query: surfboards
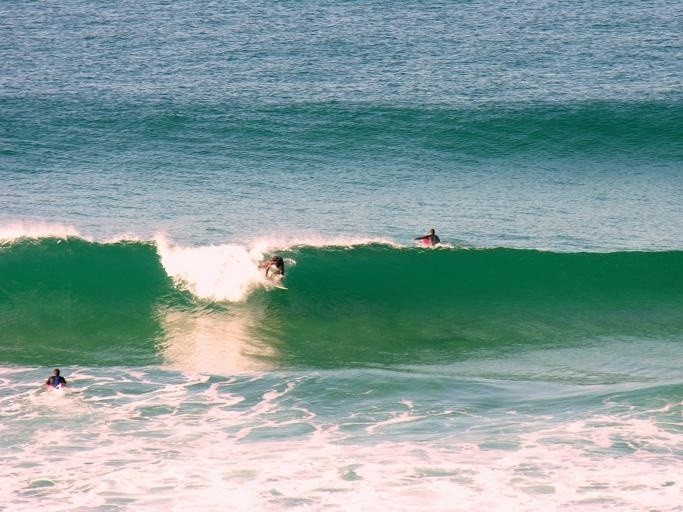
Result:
[270,280,288,290]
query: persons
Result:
[261,256,285,280]
[414,228,440,248]
[46,369,65,391]
